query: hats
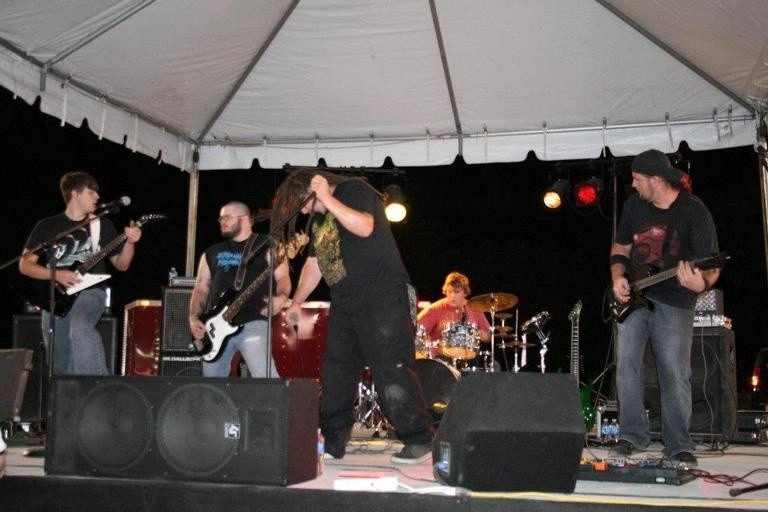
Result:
[630,149,690,184]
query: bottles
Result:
[167,265,177,286]
[317,427,325,478]
[602,418,619,438]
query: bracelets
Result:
[703,279,708,293]
[279,291,288,297]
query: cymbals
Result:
[494,332,516,337]
[496,325,513,332]
[468,293,519,313]
[495,313,512,318]
[497,341,535,347]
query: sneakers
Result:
[609,438,646,457]
[389,441,434,465]
[673,451,698,467]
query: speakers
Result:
[431,373,587,495]
[44,373,320,486]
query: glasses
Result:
[217,215,246,222]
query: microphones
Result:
[96,196,131,209]
[289,312,299,334]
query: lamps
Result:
[283,165,408,227]
[543,152,691,402]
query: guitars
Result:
[568,301,595,431]
[25,214,167,318]
[607,251,734,323]
[192,230,310,363]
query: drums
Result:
[443,323,481,360]
[414,324,430,359]
[407,283,417,331]
[413,358,461,428]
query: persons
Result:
[18,171,143,376]
[189,201,292,379]
[270,167,437,464]
[416,271,502,373]
[611,150,721,464]
